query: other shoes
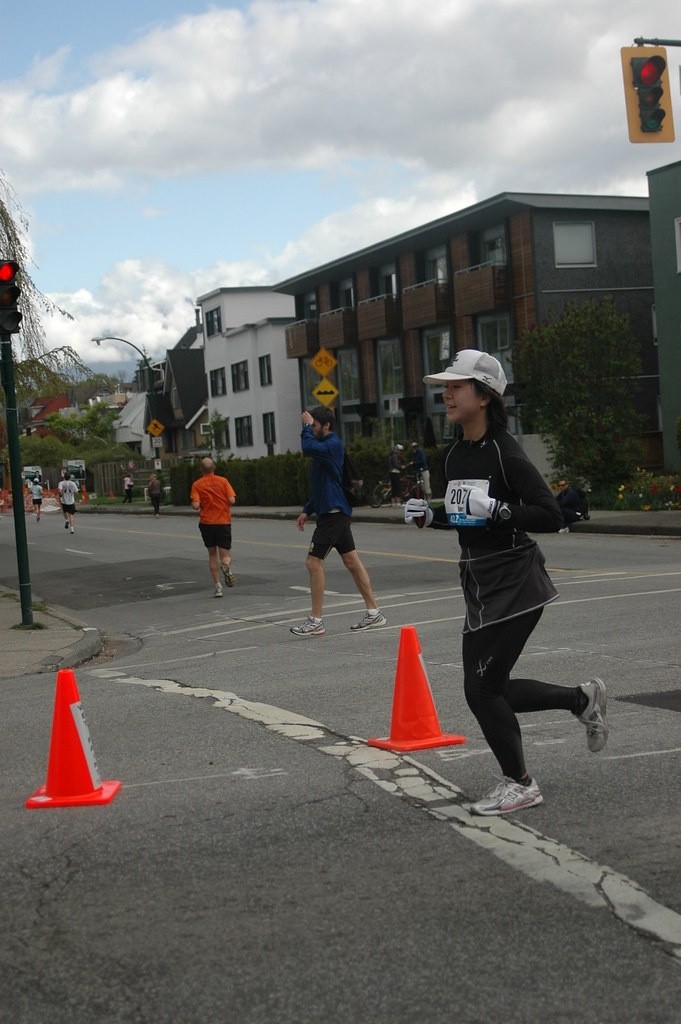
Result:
[221,561,237,588]
[214,590,224,597]
[37,514,41,522]
[71,529,74,533]
[390,503,401,507]
[65,520,69,529]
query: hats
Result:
[411,442,418,447]
[423,350,508,397]
[396,444,404,451]
[34,477,39,482]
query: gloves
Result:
[405,498,433,531]
[460,485,497,518]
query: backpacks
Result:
[332,445,362,507]
[570,486,589,521]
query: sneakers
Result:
[558,527,570,534]
[470,768,543,815]
[290,615,326,636]
[571,677,609,753]
[351,609,386,631]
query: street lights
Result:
[91,335,156,468]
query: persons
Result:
[57,472,79,534]
[555,478,582,535]
[404,354,610,816]
[148,473,161,517]
[28,478,46,522]
[290,408,386,636]
[77,465,85,478]
[407,442,432,503]
[32,471,41,482]
[190,457,236,598]
[388,443,410,508]
[122,473,134,503]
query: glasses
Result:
[558,484,566,487]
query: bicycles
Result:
[365,468,429,508]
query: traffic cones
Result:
[23,667,125,810]
[367,625,468,753]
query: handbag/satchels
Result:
[147,488,152,497]
[128,484,134,488]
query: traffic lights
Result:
[0,257,24,340]
[620,45,676,144]
